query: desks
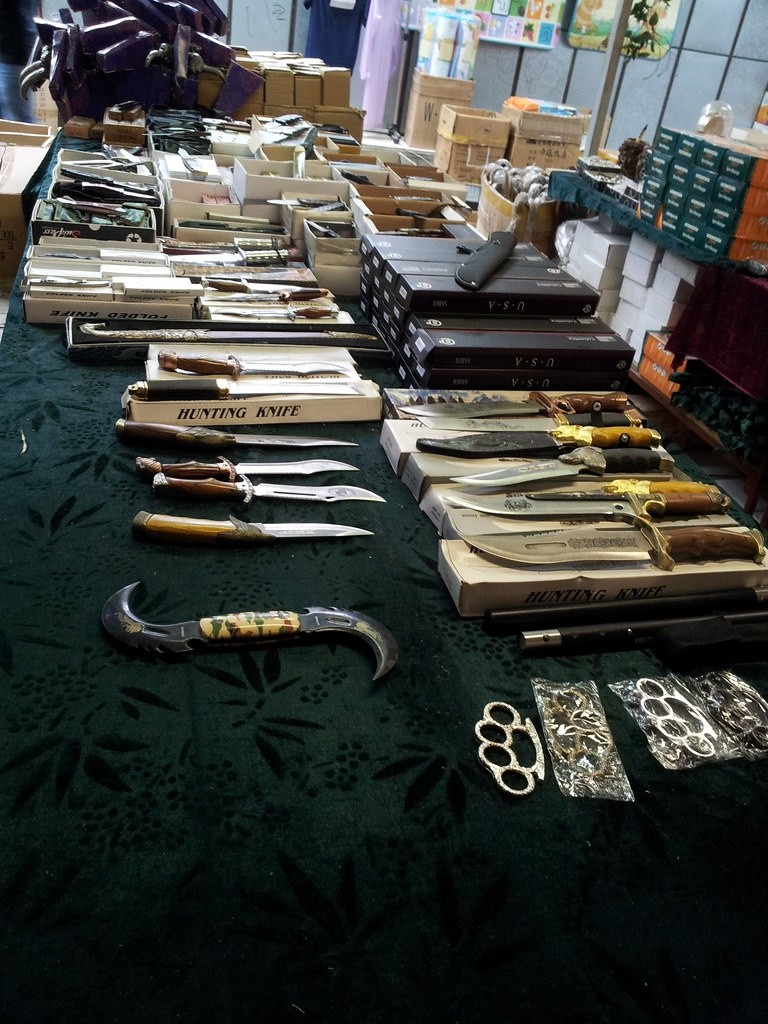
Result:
[0,129,768,1024]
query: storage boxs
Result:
[379,388,768,617]
[0,119,63,297]
[405,67,475,151]
[197,46,368,145]
[19,104,636,427]
[436,97,768,400]
